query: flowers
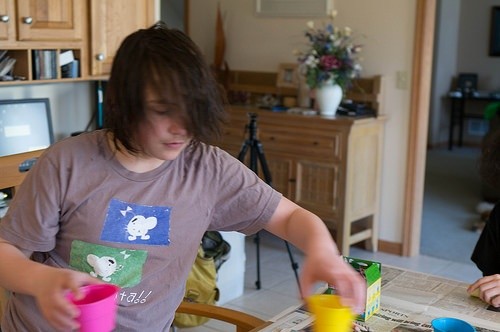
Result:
[292,10,364,91]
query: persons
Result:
[0,21,368,332]
[467,199,500,308]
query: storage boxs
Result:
[211,228,247,307]
[327,255,382,322]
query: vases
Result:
[314,71,343,120]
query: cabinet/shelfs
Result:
[0,0,157,86]
[204,70,387,258]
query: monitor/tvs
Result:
[0,98,54,158]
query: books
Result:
[33,50,56,79]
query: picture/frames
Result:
[276,63,300,89]
[254,0,329,19]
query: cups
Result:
[305,294,358,332]
[432,317,476,332]
[66,283,121,332]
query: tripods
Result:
[235,113,304,300]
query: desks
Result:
[247,263,500,332]
[447,93,500,152]
